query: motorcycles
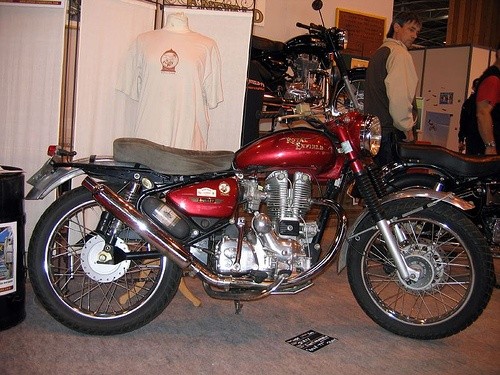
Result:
[247,0,366,118]
[25,111,496,341]
[346,134,500,259]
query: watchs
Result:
[485,141,497,147]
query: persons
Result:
[458,78,485,157]
[364,9,422,164]
[116,12,224,150]
[475,46,500,157]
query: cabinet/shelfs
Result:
[406,43,498,154]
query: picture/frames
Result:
[335,7,388,61]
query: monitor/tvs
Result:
[337,54,371,72]
